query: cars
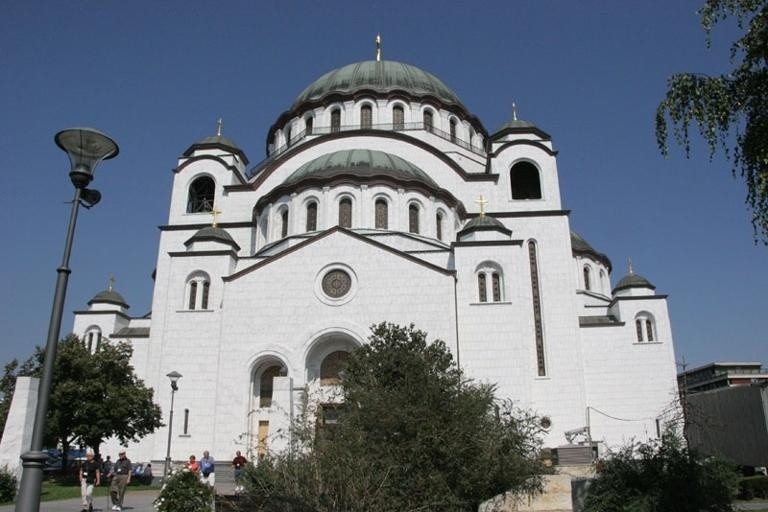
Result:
[46,449,153,485]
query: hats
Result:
[119,450,126,455]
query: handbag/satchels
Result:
[86,475,94,483]
[203,473,209,476]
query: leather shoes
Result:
[81,506,93,512]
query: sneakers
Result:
[235,485,244,491]
[112,505,120,512]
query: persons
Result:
[102,455,151,479]
[107,451,132,511]
[199,450,216,491]
[78,447,100,512]
[232,449,248,491]
[186,455,200,472]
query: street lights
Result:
[15,126,120,512]
[164,370,183,475]
